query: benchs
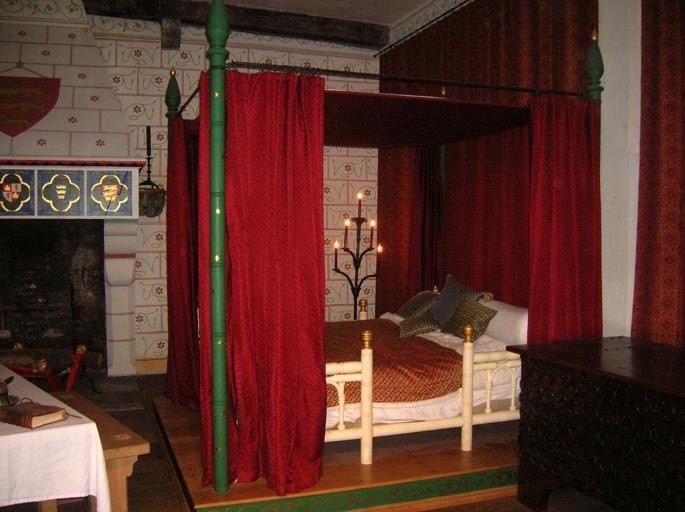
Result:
[38,389,151,512]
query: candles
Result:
[333,192,383,274]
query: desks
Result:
[1,363,112,512]
[506,335,683,511]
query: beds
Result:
[321,298,529,465]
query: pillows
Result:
[396,274,498,340]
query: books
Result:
[1,402,70,429]
